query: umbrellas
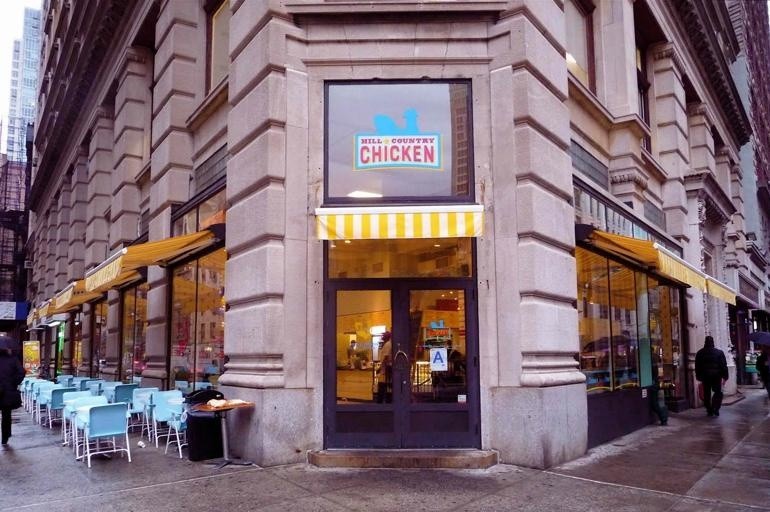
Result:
[0,336,19,349]
[583,335,679,353]
[745,330,770,346]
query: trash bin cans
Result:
[183,389,224,462]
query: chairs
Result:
[20,375,188,468]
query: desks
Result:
[193,402,255,469]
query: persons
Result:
[0,349,26,444]
[446,340,456,360]
[347,340,357,364]
[756,345,770,400]
[591,347,661,378]
[694,336,729,416]
[376,337,392,374]
